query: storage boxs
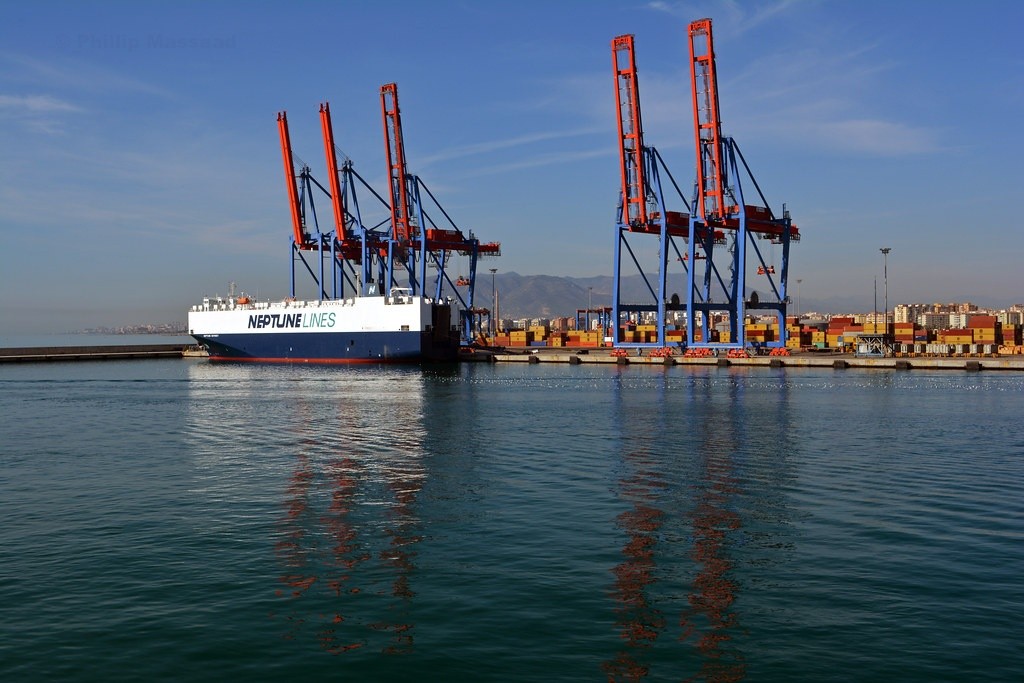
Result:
[475,317,1017,356]
[648,197,772,229]
[428,229,463,244]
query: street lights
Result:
[796,279,802,319]
[490,268,498,334]
[880,248,891,334]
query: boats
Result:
[188,287,461,364]
[182,345,210,357]
